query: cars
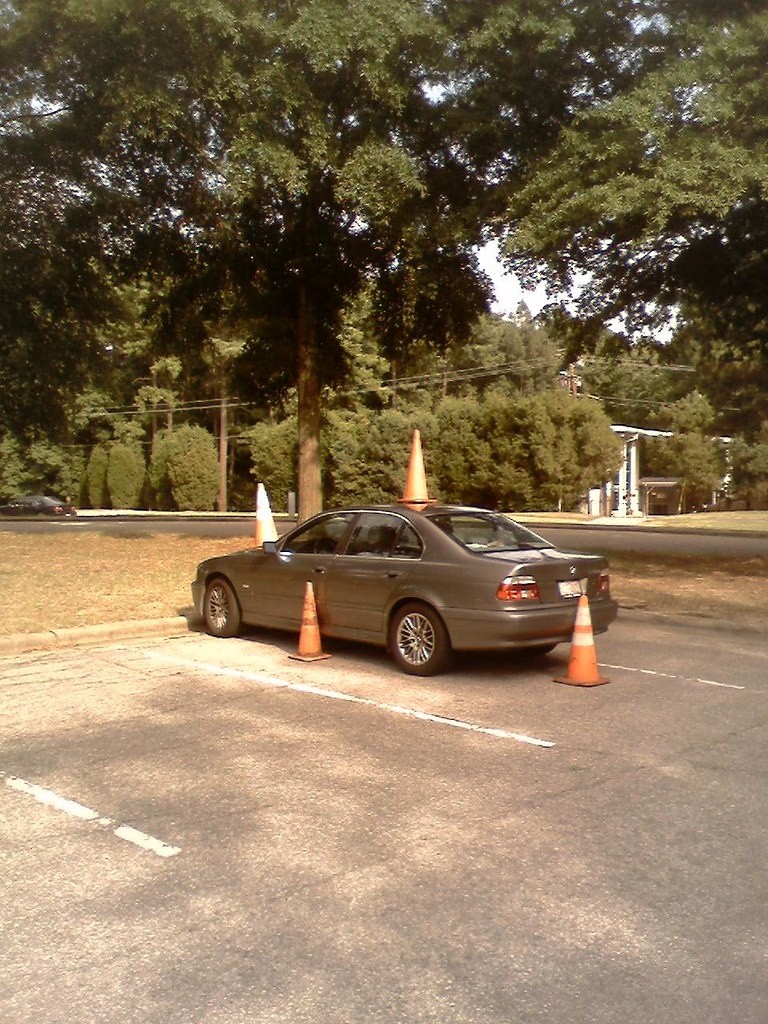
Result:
[190,504,619,676]
[0,496,77,517]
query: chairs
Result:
[367,526,397,548]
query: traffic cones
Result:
[552,594,612,687]
[287,581,333,662]
[395,429,438,503]
[253,483,280,549]
